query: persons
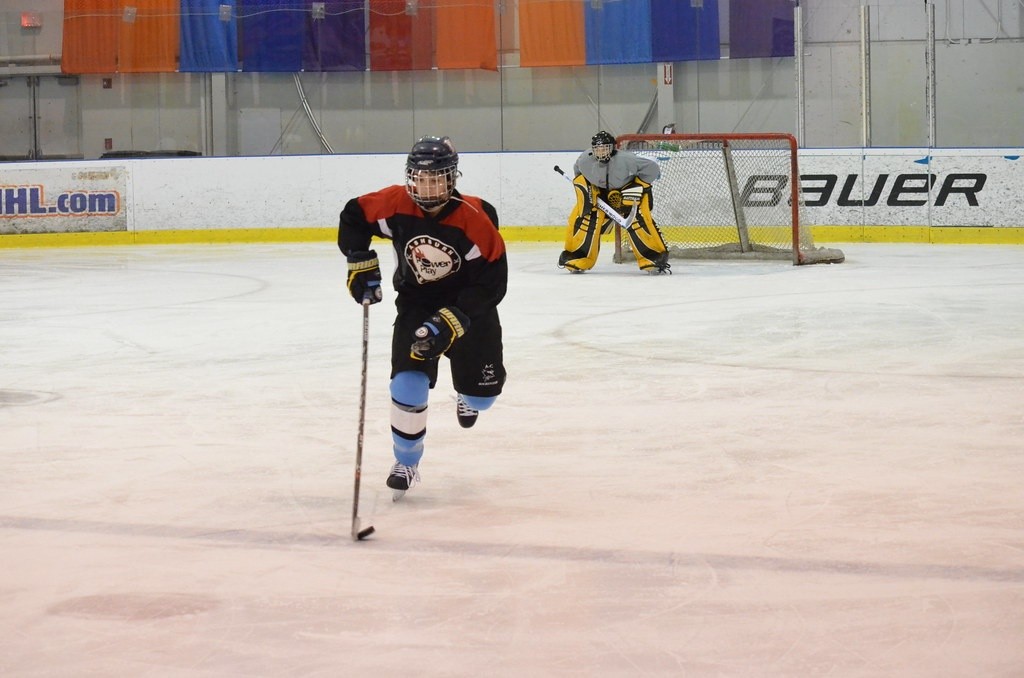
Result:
[338,136,508,501]
[559,131,670,275]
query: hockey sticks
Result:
[351,292,375,541]
[554,164,637,228]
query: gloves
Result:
[410,304,471,360]
[346,250,382,305]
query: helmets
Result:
[591,130,617,163]
[405,135,462,212]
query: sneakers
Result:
[385,460,421,502]
[456,392,479,429]
